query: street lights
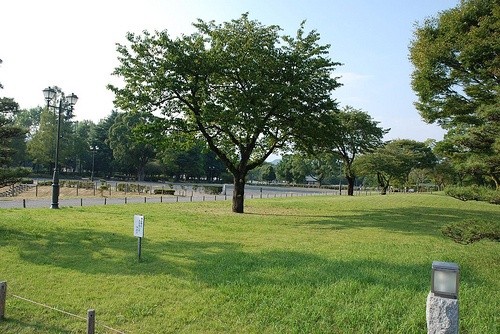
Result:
[42,87,79,210]
